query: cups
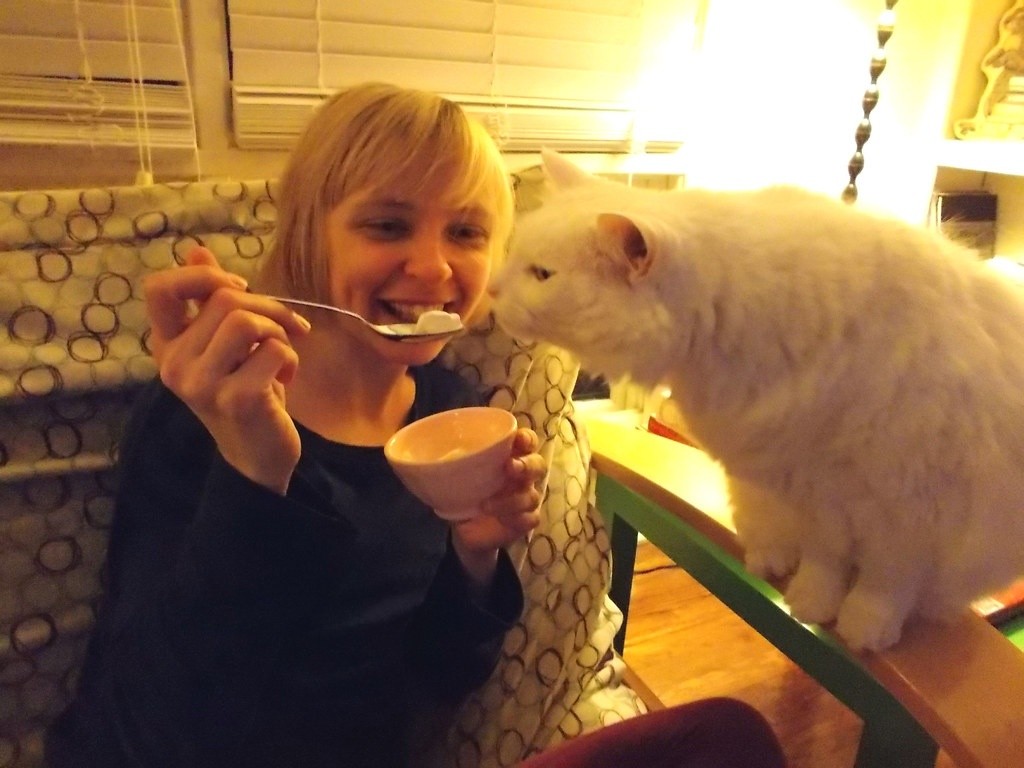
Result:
[936,190,998,261]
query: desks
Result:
[595,471,1024,768]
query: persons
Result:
[47,77,785,767]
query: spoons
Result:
[250,292,466,345]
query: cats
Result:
[488,145,1024,655]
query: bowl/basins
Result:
[384,407,518,523]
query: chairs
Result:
[0,170,1024,768]
